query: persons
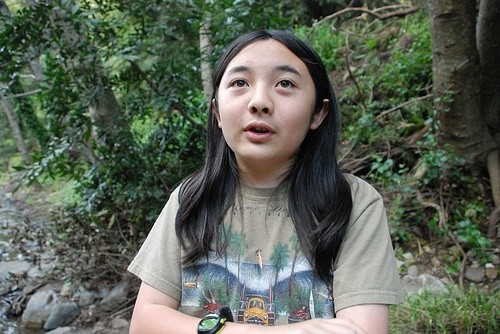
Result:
[126,30,405,334]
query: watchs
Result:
[197,310,227,334]
